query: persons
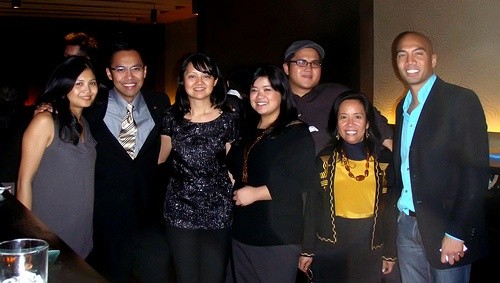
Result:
[298,90,397,283]
[16,32,314,283]
[282,40,393,160]
[393,30,490,283]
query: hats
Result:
[285,40,324,61]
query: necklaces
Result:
[242,122,274,183]
[340,144,370,181]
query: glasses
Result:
[290,58,321,68]
[111,64,145,73]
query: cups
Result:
[0,238,49,283]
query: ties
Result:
[117,104,137,160]
